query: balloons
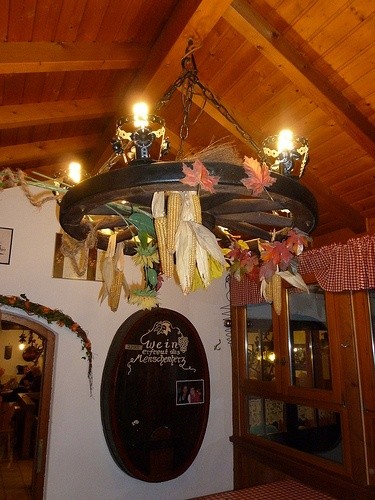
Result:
[23,346,38,362]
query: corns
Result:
[106,188,285,317]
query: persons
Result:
[0,356,44,461]
[189,387,200,403]
[178,385,191,404]
[197,387,203,399]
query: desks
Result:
[199,479,337,500]
